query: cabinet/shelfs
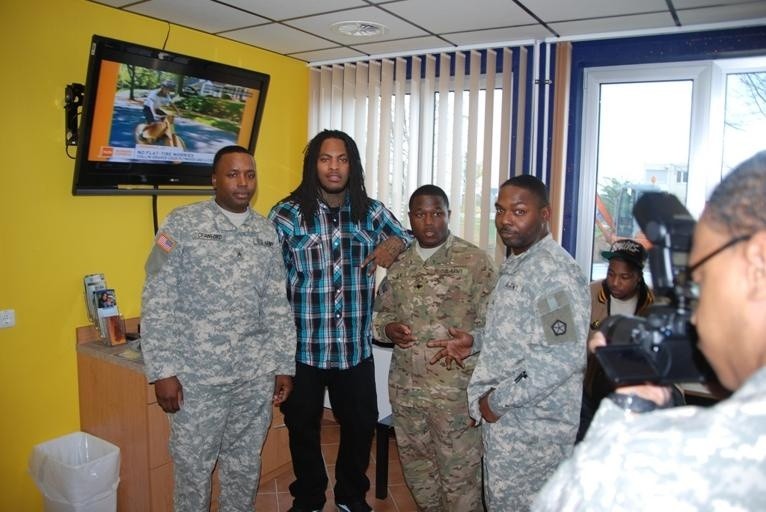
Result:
[76,317,292,512]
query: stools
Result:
[375,412,394,500]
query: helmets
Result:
[160,79,176,90]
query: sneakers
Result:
[334,493,373,512]
[286,503,323,512]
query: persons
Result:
[143,81,176,126]
[424,174,592,512]
[140,146,298,512]
[531,152,766,512]
[99,292,115,308]
[370,184,499,512]
[267,129,413,511]
[574,240,655,451]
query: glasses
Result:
[671,233,755,300]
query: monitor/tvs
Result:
[72,34,270,196]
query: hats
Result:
[600,239,650,267]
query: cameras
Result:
[593,192,707,387]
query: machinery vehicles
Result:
[595,182,663,263]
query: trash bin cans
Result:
[33,431,120,512]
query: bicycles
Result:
[131,107,187,166]
